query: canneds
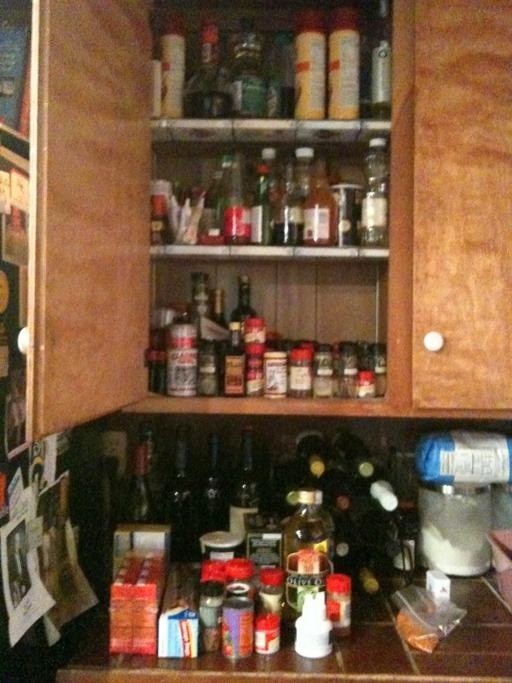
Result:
[223,583,254,660]
[263,342,314,399]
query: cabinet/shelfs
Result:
[22,0,510,444]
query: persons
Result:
[8,530,28,607]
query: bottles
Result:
[293,590,336,660]
[286,424,401,638]
[153,0,393,119]
[151,270,265,396]
[150,193,172,245]
[199,579,225,654]
[230,425,265,515]
[202,137,389,248]
[162,424,199,561]
[264,337,388,399]
[258,570,283,623]
[197,428,230,532]
[124,419,163,522]
[257,439,289,515]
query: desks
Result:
[53,562,512,680]
